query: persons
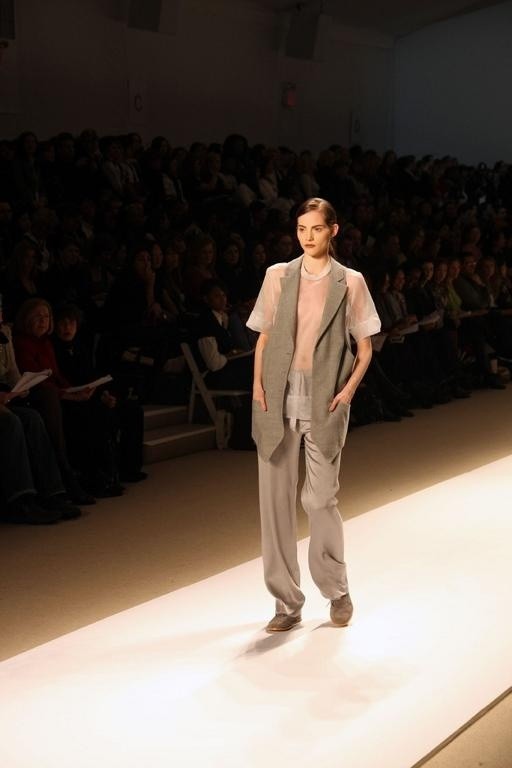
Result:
[0,129,512,528]
[242,195,383,633]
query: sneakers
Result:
[5,462,147,527]
[266,612,303,631]
[346,353,512,424]
[329,590,354,627]
